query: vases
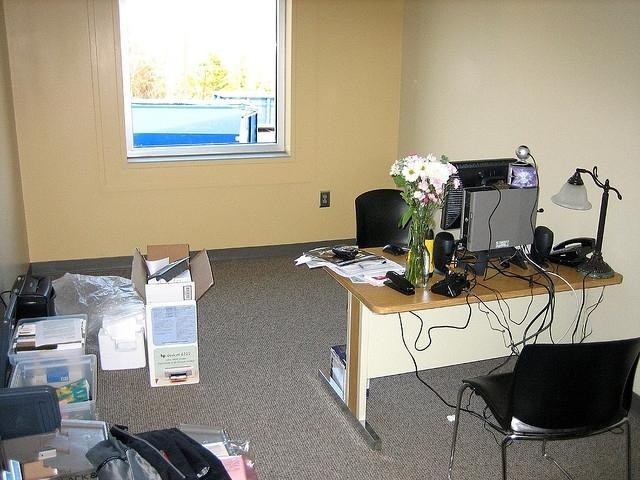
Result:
[404,233,426,288]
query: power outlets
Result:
[320,191,331,207]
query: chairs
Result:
[445,336,640,479]
[353,189,414,253]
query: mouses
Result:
[383,244,404,256]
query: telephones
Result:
[548,238,595,266]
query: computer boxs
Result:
[461,185,538,253]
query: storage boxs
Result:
[178,422,237,460]
[130,241,215,388]
[10,355,101,421]
[7,313,88,360]
[0,420,112,479]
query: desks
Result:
[311,242,624,453]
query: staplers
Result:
[384,271,415,295]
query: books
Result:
[296,245,405,289]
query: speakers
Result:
[532,225,553,269]
[432,232,454,274]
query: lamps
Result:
[550,162,621,279]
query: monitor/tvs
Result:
[440,158,534,276]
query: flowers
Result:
[387,151,459,282]
